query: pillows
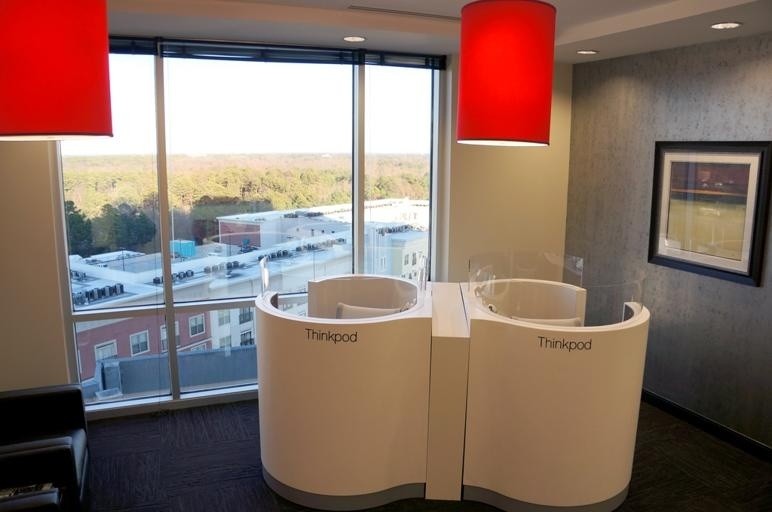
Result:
[487,303,583,327]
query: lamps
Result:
[455,0,557,148]
[0,1,115,142]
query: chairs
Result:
[0,382,90,511]
[334,301,409,319]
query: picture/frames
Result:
[646,138,771,287]
